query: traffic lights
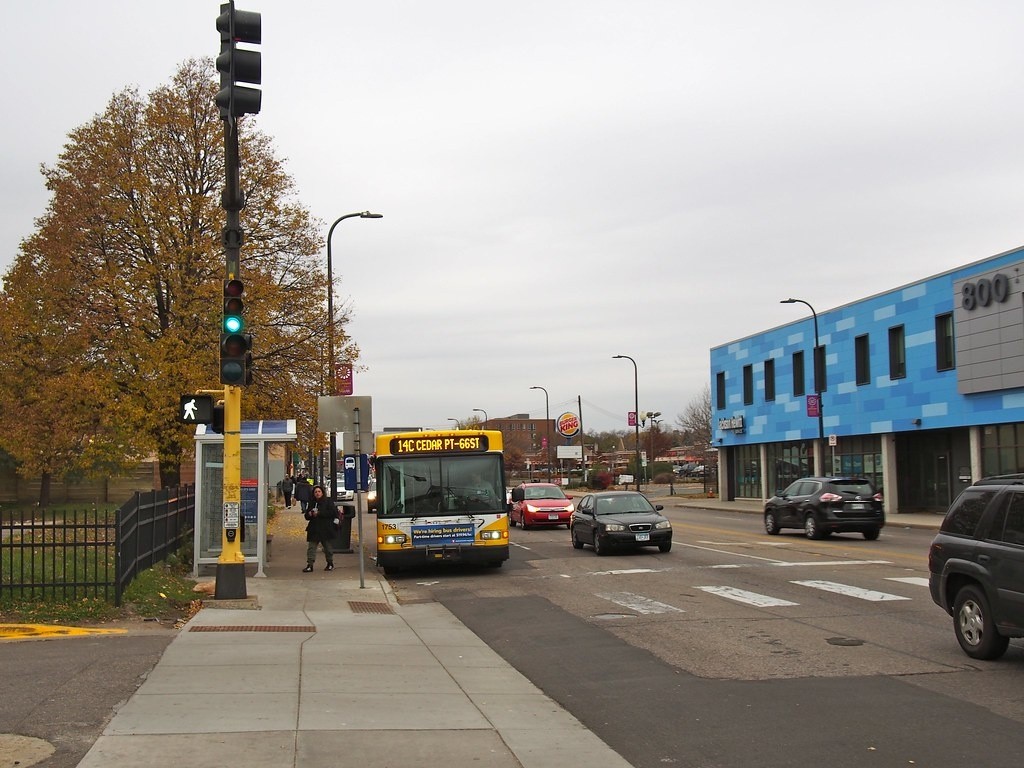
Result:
[218,333,253,385]
[222,279,244,334]
[178,394,213,424]
[214,0,262,116]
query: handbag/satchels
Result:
[292,498,296,506]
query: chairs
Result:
[527,489,532,498]
[597,500,610,513]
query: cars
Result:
[571,491,673,556]
[319,471,377,501]
[507,481,574,530]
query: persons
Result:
[462,471,497,510]
[420,462,459,511]
[282,473,294,510]
[303,486,339,572]
[290,474,311,513]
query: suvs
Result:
[764,477,886,540]
[928,473,1024,660]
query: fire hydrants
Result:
[669,483,676,495]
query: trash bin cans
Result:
[322,502,355,554]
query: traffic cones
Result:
[707,487,714,498]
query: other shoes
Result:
[288,506,291,509]
[302,510,306,513]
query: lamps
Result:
[715,437,721,442]
[911,419,922,425]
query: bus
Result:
[373,429,525,575]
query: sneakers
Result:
[325,562,333,572]
[302,564,313,572]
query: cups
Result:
[313,508,318,517]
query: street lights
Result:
[647,411,661,481]
[529,386,550,484]
[473,408,488,429]
[780,298,825,477]
[327,211,383,503]
[611,354,647,492]
